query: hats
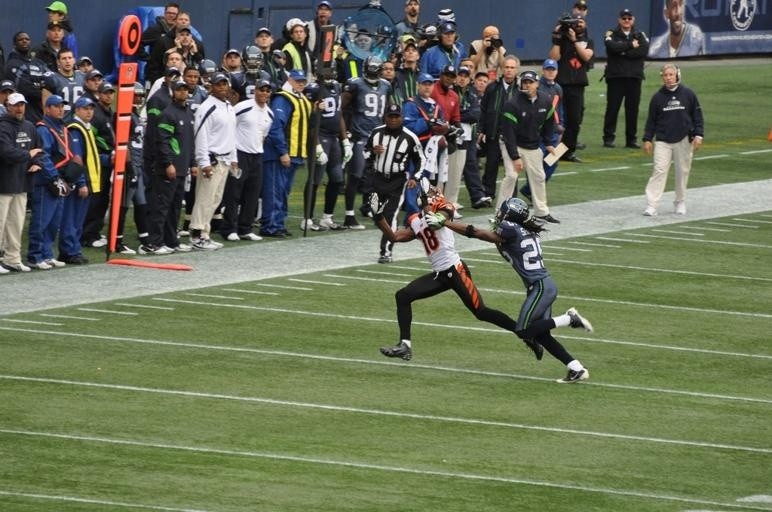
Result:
[571,14,586,21]
[0,1,116,108]
[574,0,589,10]
[383,104,402,117]
[520,70,539,83]
[542,59,559,70]
[162,0,490,110]
[619,8,634,17]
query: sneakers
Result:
[1,261,31,272]
[3,266,10,274]
[566,307,593,332]
[560,155,582,163]
[471,195,495,209]
[300,219,328,231]
[557,366,591,384]
[57,253,89,265]
[227,232,240,241]
[43,258,66,267]
[379,341,414,361]
[176,227,190,239]
[520,189,532,202]
[318,216,348,231]
[576,143,587,150]
[523,339,544,360]
[239,232,263,240]
[626,140,641,149]
[25,260,53,270]
[674,201,686,215]
[189,238,224,251]
[453,202,466,220]
[115,245,137,255]
[172,243,192,252]
[604,141,615,148]
[91,235,108,247]
[137,244,176,255]
[531,214,561,225]
[642,204,659,216]
[344,215,366,230]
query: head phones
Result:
[516,70,539,88]
[659,63,681,81]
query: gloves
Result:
[425,210,446,230]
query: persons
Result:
[603,9,650,149]
[368,185,544,361]
[1,0,595,275]
[426,199,593,384]
[650,0,706,58]
[642,64,704,216]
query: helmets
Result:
[418,184,447,208]
[497,197,530,224]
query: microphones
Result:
[670,81,680,86]
[518,87,533,93]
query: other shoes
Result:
[367,210,374,223]
[257,229,293,237]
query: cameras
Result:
[486,38,504,47]
